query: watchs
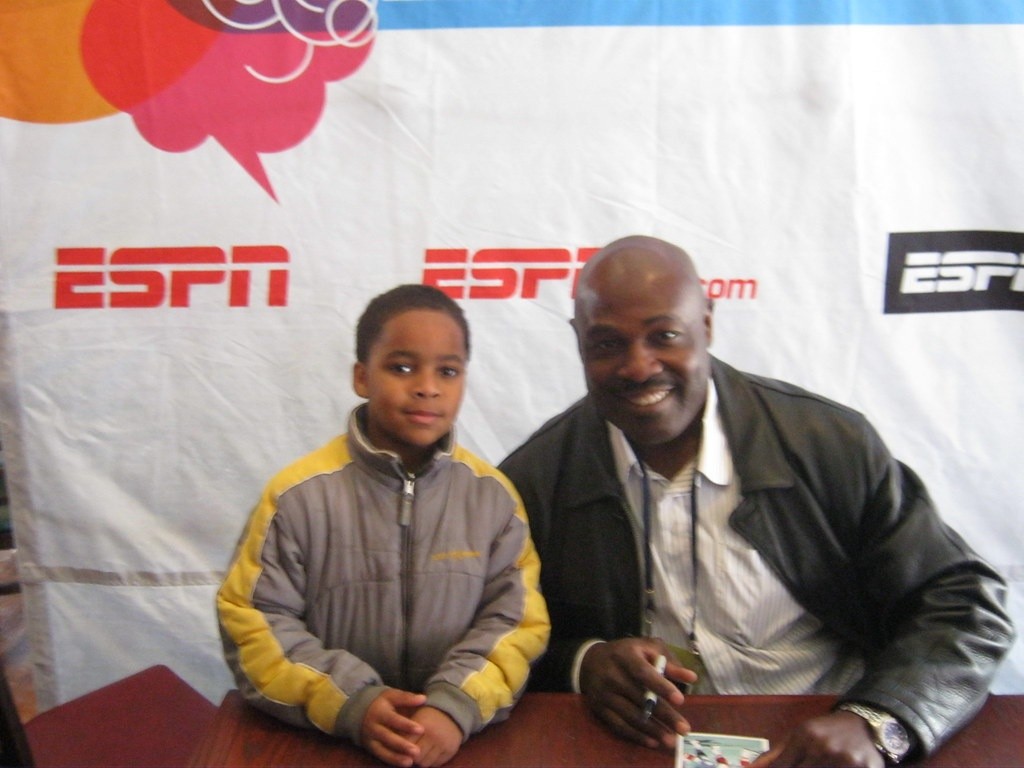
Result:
[836,701,915,768]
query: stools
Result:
[20,665,218,768]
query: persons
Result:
[215,285,551,767]
[495,234,1014,768]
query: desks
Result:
[193,689,1023,768]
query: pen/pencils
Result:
[637,656,665,728]
